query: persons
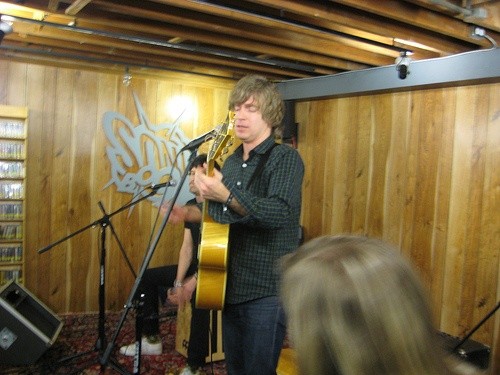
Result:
[118,74,306,375]
[275,233,457,375]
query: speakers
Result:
[0,279,64,370]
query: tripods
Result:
[39,190,156,375]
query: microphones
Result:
[151,179,176,188]
[182,125,223,151]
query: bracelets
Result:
[173,280,184,288]
[224,191,233,207]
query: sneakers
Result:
[120,336,162,355]
[180,363,201,375]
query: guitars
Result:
[193,109,239,313]
